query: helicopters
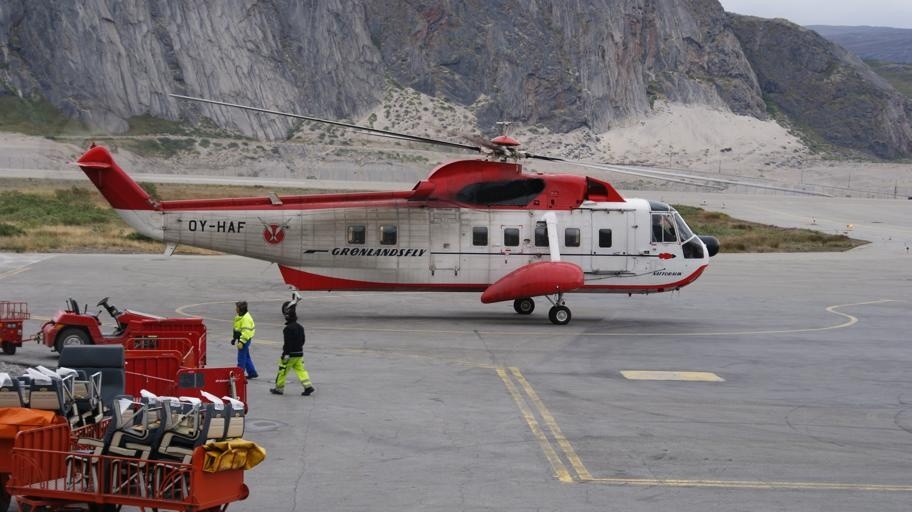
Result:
[66,94,831,324]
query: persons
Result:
[270,309,314,395]
[652,215,674,242]
[231,301,258,379]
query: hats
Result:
[285,310,297,321]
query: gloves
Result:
[237,341,243,349]
[283,355,290,361]
[230,339,235,345]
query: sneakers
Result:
[269,387,284,395]
[301,386,315,396]
[245,375,261,380]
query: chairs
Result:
[69,295,99,320]
[0,344,246,499]
